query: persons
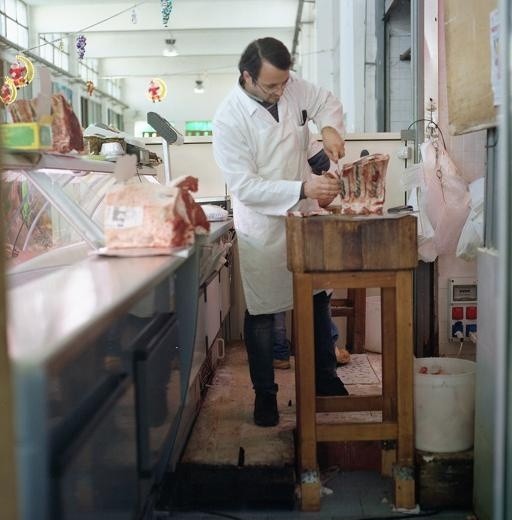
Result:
[210,34,355,426]
[6,53,36,89]
[146,78,167,104]
[0,76,19,105]
[272,125,363,370]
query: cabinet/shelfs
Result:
[1,152,158,294]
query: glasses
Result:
[253,73,294,95]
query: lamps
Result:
[193,74,205,98]
[162,29,180,57]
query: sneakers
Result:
[317,378,349,397]
[274,359,290,369]
[336,348,350,364]
[253,394,279,427]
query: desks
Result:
[290,267,419,511]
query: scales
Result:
[146,111,229,222]
